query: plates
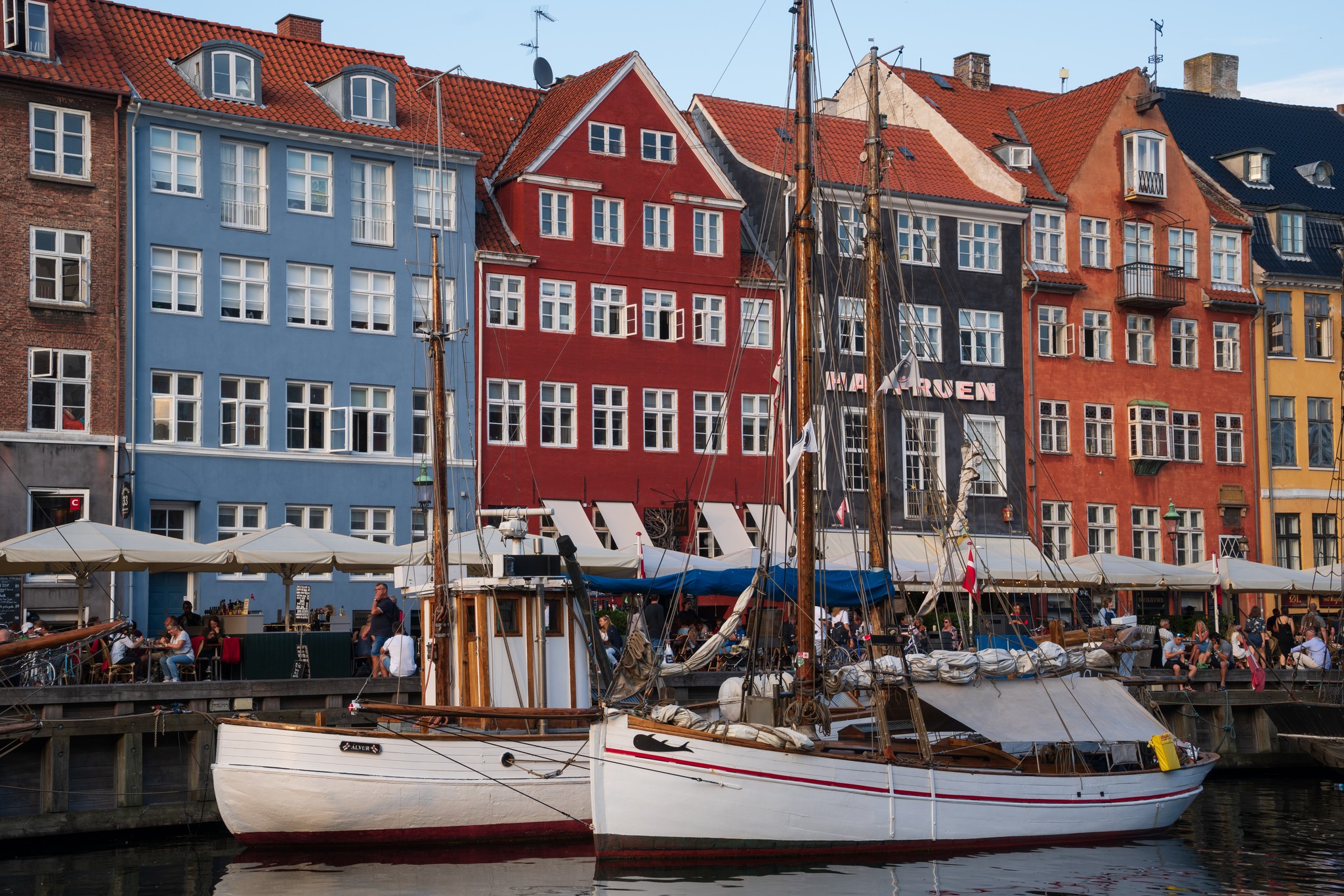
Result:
[153,645,165,647]
[138,645,147,647]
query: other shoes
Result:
[162,679,179,682]
[127,680,136,683]
[1285,665,1293,669]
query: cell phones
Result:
[1215,639,1220,645]
[1183,649,1187,652]
[209,632,214,636]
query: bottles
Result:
[737,634,740,640]
[326,612,331,622]
[289,611,292,623]
[703,628,707,640]
[742,634,744,639]
[791,658,794,671]
[735,628,737,640]
[693,629,696,640]
[340,606,345,616]
[309,607,327,623]
[208,599,244,615]
[339,608,341,616]
[312,614,320,631]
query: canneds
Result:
[933,625,936,631]
[1184,660,1188,666]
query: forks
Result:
[141,636,146,644]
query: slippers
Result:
[1218,685,1226,691]
[1180,689,1185,691]
[1187,689,1198,692]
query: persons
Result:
[900,615,927,654]
[1162,631,1198,692]
[0,601,224,687]
[1224,624,1246,669]
[1098,597,1115,626]
[1244,602,1344,670]
[323,583,405,677]
[1008,602,1028,624]
[627,592,744,672]
[780,604,872,666]
[1158,618,1174,648]
[1188,620,1209,668]
[1201,632,1235,691]
[593,614,622,673]
[380,621,417,677]
[942,616,959,651]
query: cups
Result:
[282,616,285,624]
[711,631,713,635]
[933,625,936,631]
[148,638,154,647]
[707,632,711,635]
[150,638,155,645]
[1183,635,1186,640]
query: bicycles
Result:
[903,617,929,655]
[826,625,873,666]
[950,619,976,651]
[0,624,83,687]
[1023,617,1089,636]
[793,618,851,670]
[588,615,625,673]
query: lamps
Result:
[426,642,438,660]
[1231,525,1251,553]
[1002,502,1014,522]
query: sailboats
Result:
[586,0,1221,866]
[207,63,1147,848]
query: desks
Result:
[202,643,223,682]
[263,622,331,632]
[135,643,172,683]
[695,639,709,646]
[1180,638,1197,662]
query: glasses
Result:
[943,621,949,624]
[374,588,385,592]
[1196,623,1204,626]
[1176,633,1184,637]
[167,627,174,632]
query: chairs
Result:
[98,638,135,685]
[671,633,729,672]
[210,636,245,681]
[1223,635,1344,669]
[144,659,165,683]
[410,636,421,676]
[174,635,206,682]
[84,640,118,685]
[1154,636,1169,669]
[349,628,372,677]
[758,636,794,672]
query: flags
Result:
[1241,634,1265,692]
[962,542,980,602]
[1211,550,1223,606]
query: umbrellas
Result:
[1052,549,1221,609]
[913,546,1099,615]
[165,522,423,632]
[1179,556,1344,633]
[710,546,858,571]
[398,525,642,579]
[821,550,964,584]
[0,519,230,685]
[584,541,748,578]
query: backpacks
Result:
[1303,615,1319,636]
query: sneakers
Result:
[205,667,212,673]
[207,674,211,678]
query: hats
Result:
[21,622,34,633]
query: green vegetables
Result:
[155,644,163,645]
[139,643,146,645]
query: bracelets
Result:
[1216,649,1220,653]
[1331,640,1334,642]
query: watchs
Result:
[1288,654,1292,657]
[373,598,379,602]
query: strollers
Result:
[720,636,754,671]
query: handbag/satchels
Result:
[662,643,674,663]
[835,621,845,630]
[158,650,178,658]
[124,650,136,657]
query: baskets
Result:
[832,626,849,645]
[597,624,608,641]
[966,625,980,639]
[915,631,929,647]
[961,639,976,648]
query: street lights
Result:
[411,458,433,541]
